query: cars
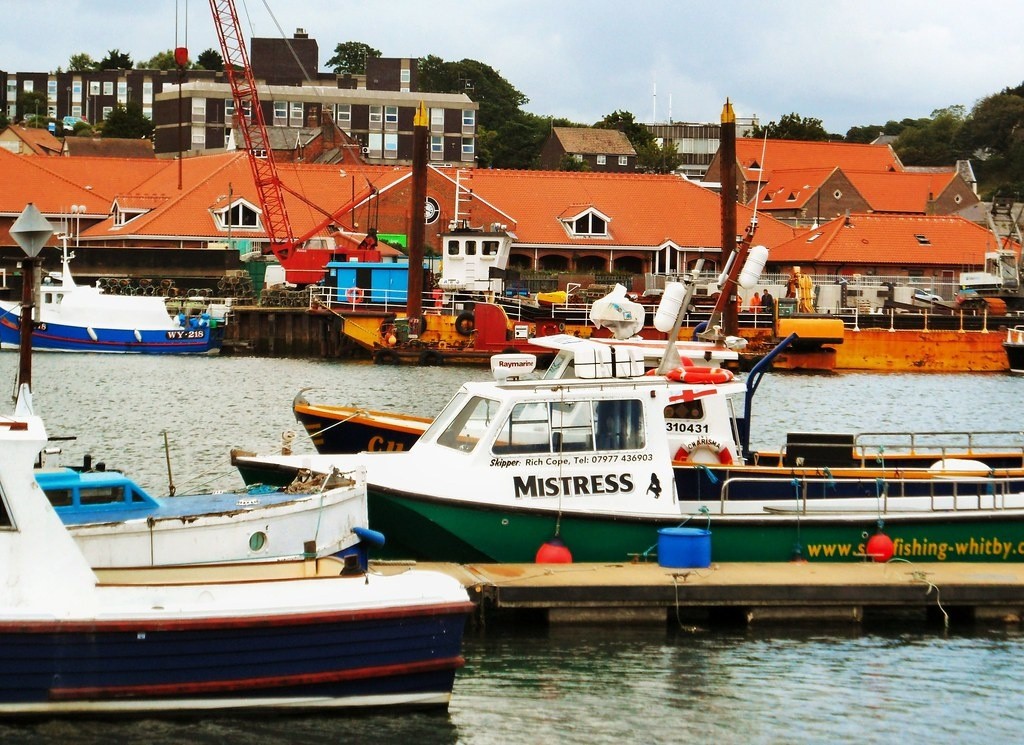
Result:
[912,287,945,301]
[62,117,90,127]
[48,122,73,133]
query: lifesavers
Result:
[346,286,363,303]
[673,435,735,466]
[454,313,477,337]
[668,365,735,384]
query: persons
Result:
[762,290,775,314]
[748,292,763,314]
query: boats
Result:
[229,372,1024,565]
[1002,325,1024,375]
[280,380,1024,492]
[0,291,231,357]
[0,204,478,719]
[586,332,740,378]
[31,442,372,576]
[28,223,103,300]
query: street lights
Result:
[70,204,85,246]
[715,96,742,340]
[397,96,430,325]
[34,97,40,129]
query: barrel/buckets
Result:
[657,507,712,568]
